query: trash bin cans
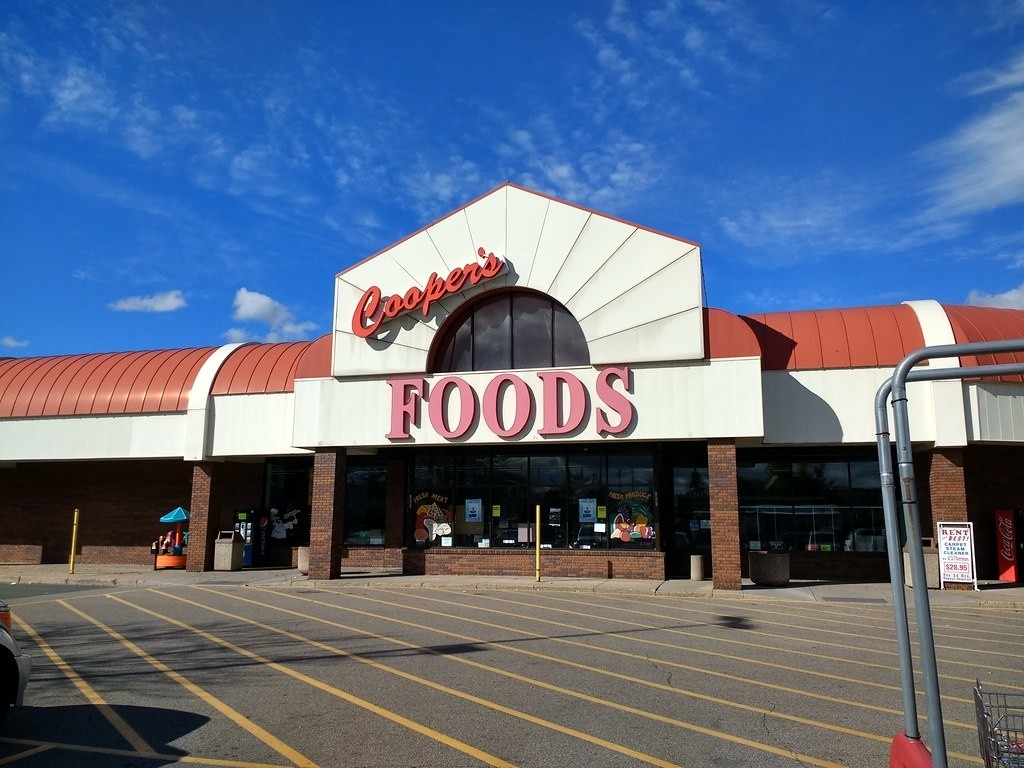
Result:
[211,529,245,571]
[901,537,939,589]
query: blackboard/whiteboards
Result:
[936,521,978,583]
